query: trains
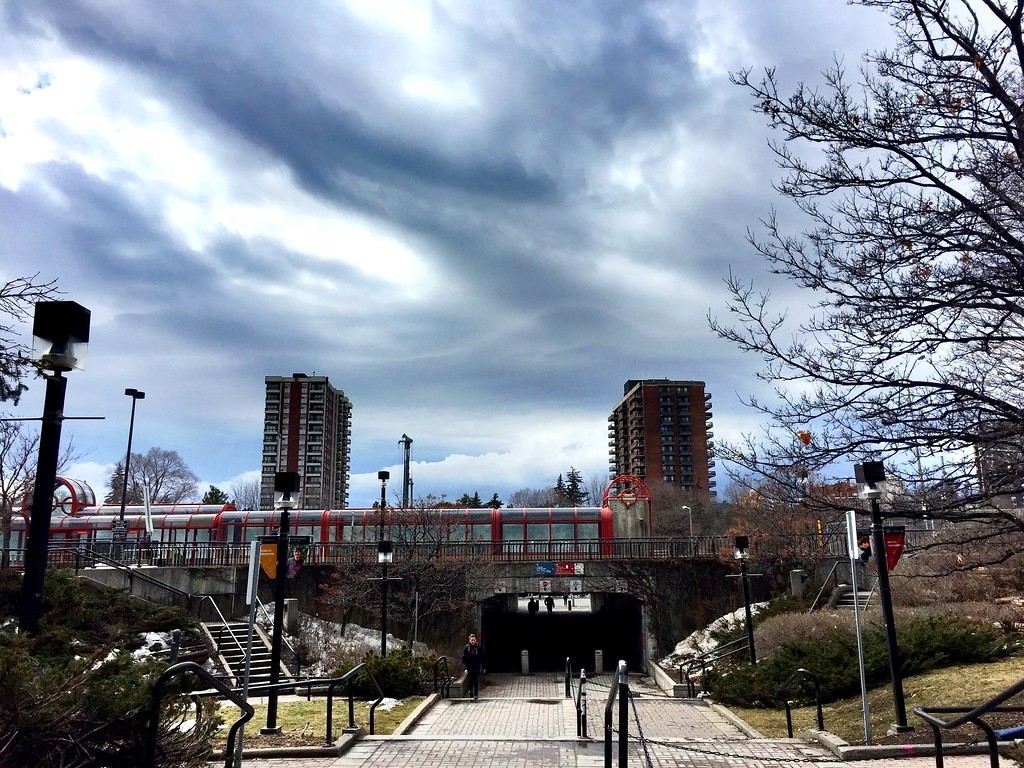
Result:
[0,502,613,571]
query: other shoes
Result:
[474,690,478,699]
[470,691,473,698]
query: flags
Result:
[884,525,905,569]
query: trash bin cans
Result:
[254,534,280,563]
[288,535,314,565]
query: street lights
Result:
[855,460,916,734]
[378,471,391,541]
[681,505,694,556]
[378,540,394,658]
[259,471,302,734]
[17,299,93,637]
[733,535,757,664]
[118,388,146,521]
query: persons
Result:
[288,545,303,578]
[461,633,486,702]
[545,594,554,613]
[861,537,872,559]
[563,592,569,604]
[528,597,538,614]
